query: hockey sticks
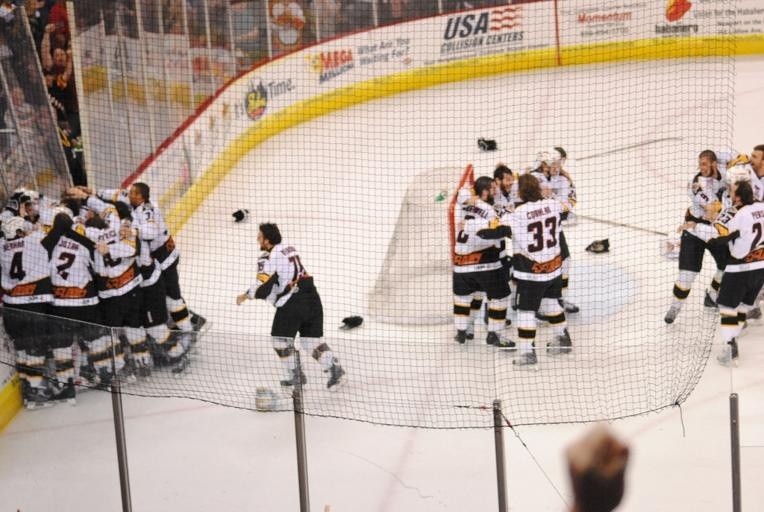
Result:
[575,136,682,161]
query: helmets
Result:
[722,166,751,184]
[534,148,560,178]
[42,206,74,228]
[4,216,33,240]
[7,188,43,216]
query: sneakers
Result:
[665,306,679,323]
[512,352,536,364]
[704,293,718,308]
[280,368,307,385]
[717,338,738,361]
[564,302,578,314]
[544,335,570,352]
[79,366,153,385]
[455,314,514,348]
[327,366,345,388]
[173,314,207,371]
[23,383,75,402]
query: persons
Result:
[208,0,514,79]
[236,223,346,393]
[0,0,208,410]
[449,146,580,366]
[565,426,628,512]
[663,145,764,367]
